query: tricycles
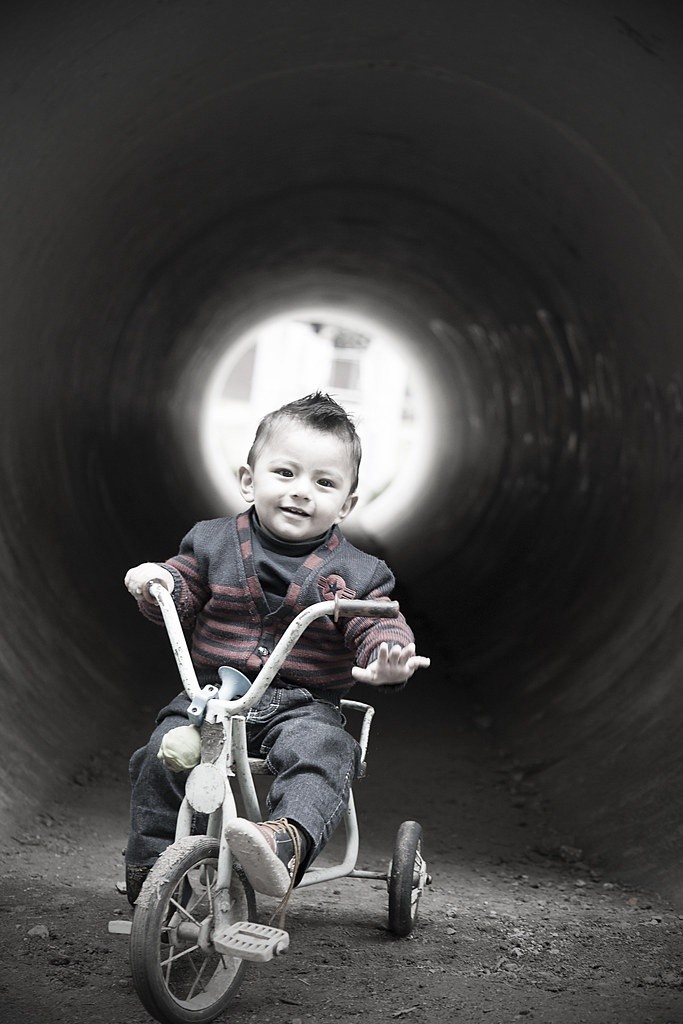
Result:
[105,573,433,1024]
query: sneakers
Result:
[160,919,180,948]
[225,817,306,897]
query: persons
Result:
[124,387,429,940]
